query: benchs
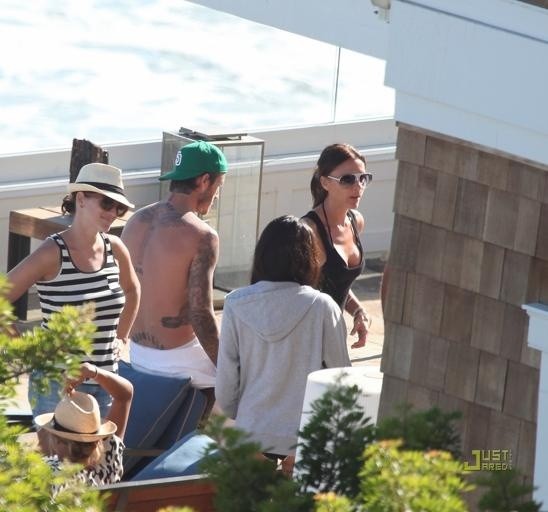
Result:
[85,474,223,512]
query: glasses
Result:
[327,173,373,186]
[85,192,128,217]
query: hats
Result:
[159,140,228,181]
[34,391,118,443]
[67,162,135,209]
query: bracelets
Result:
[353,306,363,314]
[346,295,355,305]
[89,367,99,381]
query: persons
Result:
[114,138,231,460]
[214,212,351,482]
[21,362,134,511]
[0,162,143,430]
[296,140,372,352]
